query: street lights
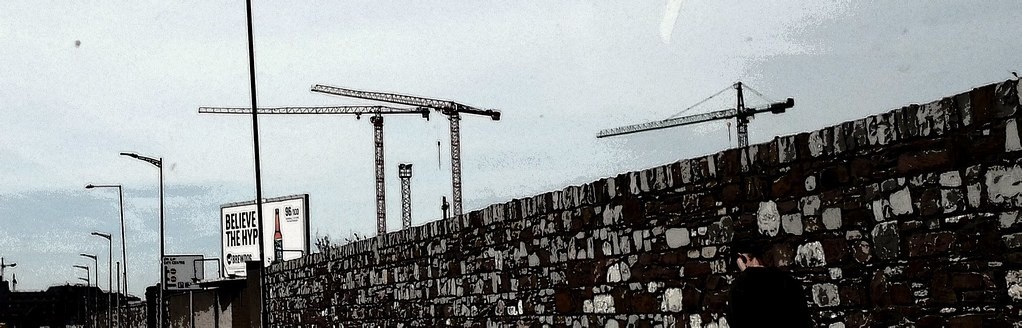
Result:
[122,152,166,328]
[85,185,132,328]
[92,232,113,328]
[73,253,98,328]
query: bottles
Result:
[274,208,283,263]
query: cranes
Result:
[595,81,793,148]
[199,105,430,233]
[0,257,19,280]
[312,84,502,216]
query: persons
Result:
[726,232,814,328]
